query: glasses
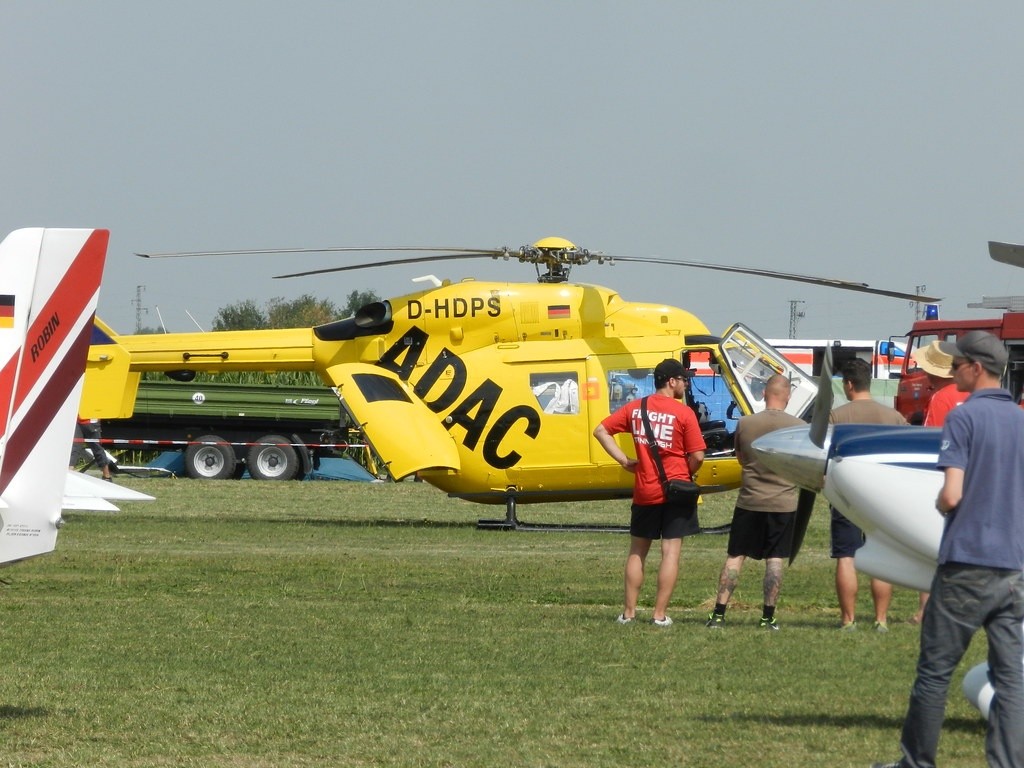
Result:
[677,377,688,382]
[950,360,973,368]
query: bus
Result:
[687,336,921,380]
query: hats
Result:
[939,330,1007,373]
[911,340,954,379]
[654,358,697,380]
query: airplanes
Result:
[749,339,996,721]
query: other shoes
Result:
[870,761,903,768]
[873,621,890,632]
[839,619,857,629]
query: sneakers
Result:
[616,613,635,624]
[759,616,780,630]
[650,615,673,627]
[706,612,726,629]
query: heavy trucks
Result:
[97,377,356,482]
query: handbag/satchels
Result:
[666,480,700,507]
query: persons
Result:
[909,339,970,626]
[868,331,1024,768]
[706,373,808,629]
[592,358,705,626]
[827,357,910,632]
[68,419,113,483]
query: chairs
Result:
[684,352,729,443]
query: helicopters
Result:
[72,235,943,537]
